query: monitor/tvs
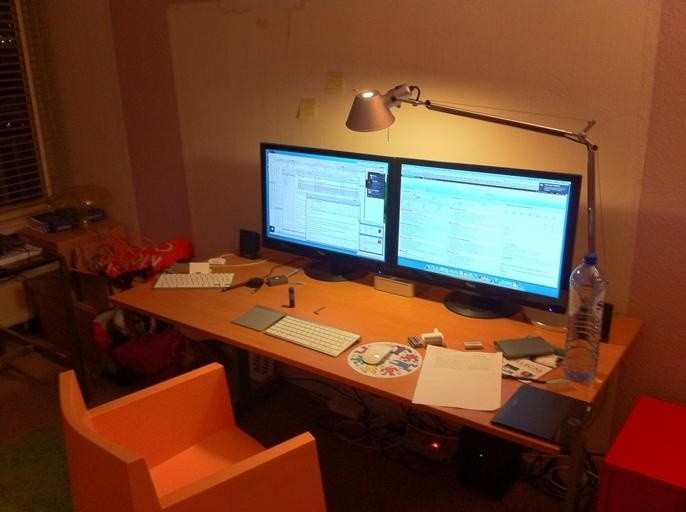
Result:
[259,140,390,283]
[390,156,584,320]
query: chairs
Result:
[60,360,336,511]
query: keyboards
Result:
[264,313,361,358]
[152,272,234,290]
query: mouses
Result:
[361,343,391,366]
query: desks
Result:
[109,256,644,506]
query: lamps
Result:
[343,81,614,345]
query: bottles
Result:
[563,254,605,383]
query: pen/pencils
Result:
[502,375,547,384]
[223,283,245,291]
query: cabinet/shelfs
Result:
[16,221,124,349]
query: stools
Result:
[594,395,686,511]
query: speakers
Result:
[574,298,614,344]
[238,228,261,259]
